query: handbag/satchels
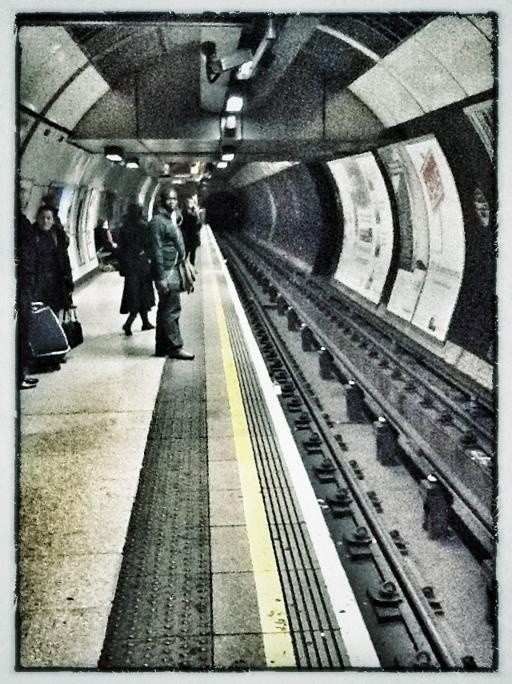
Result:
[157,213,198,294]
[59,307,85,349]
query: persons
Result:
[16,184,206,389]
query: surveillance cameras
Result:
[211,48,253,74]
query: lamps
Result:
[103,93,244,183]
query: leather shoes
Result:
[157,348,194,361]
[122,324,132,336]
[141,324,155,330]
[24,377,39,384]
[20,381,36,388]
